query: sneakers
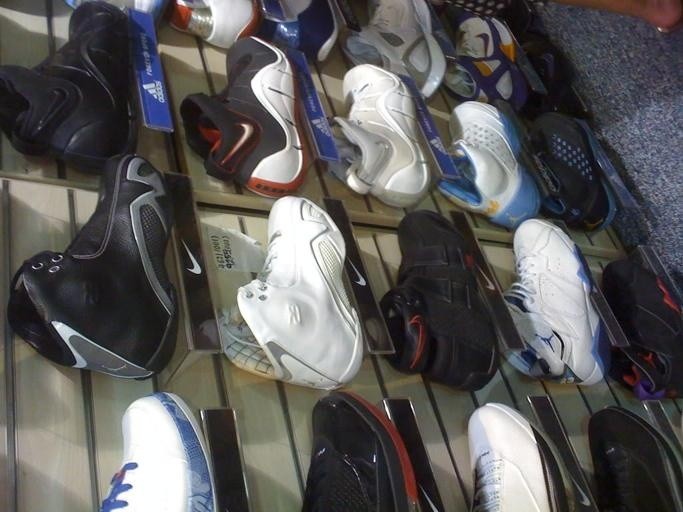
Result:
[8,154,177,379]
[466,399,574,511]
[103,392,217,511]
[301,389,419,512]
[587,404,683,512]
[2,0,164,166]
